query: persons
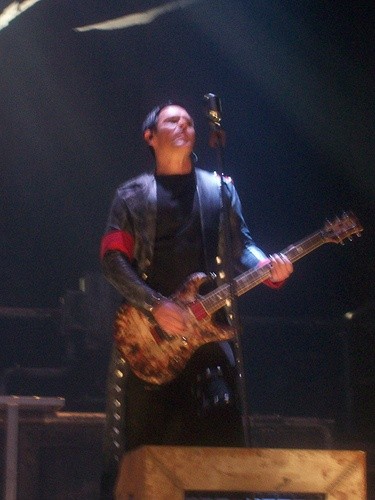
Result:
[99,103,295,463]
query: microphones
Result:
[203,93,222,118]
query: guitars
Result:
[111,210,364,386]
